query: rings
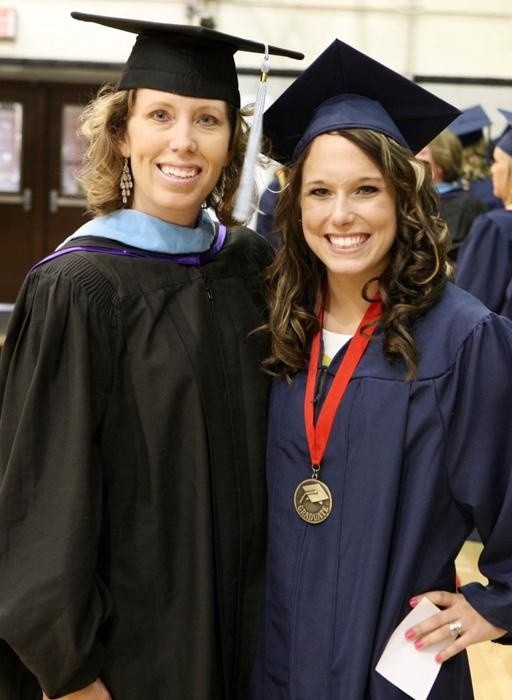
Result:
[447,620,463,639]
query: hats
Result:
[70,12,304,108]
[260,38,462,167]
[448,106,512,154]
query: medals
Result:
[293,475,334,526]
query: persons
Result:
[257,166,293,251]
[0,11,311,697]
[257,38,511,700]
[448,100,502,213]
[448,108,512,321]
[416,125,488,266]
